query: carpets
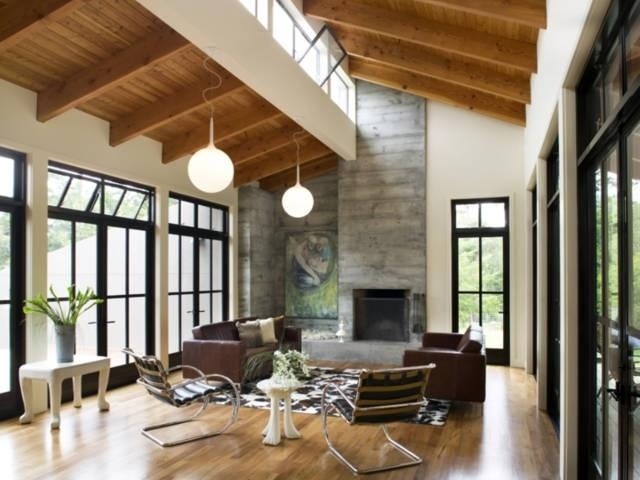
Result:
[209,365,453,427]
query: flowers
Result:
[271,350,312,379]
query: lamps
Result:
[187,56,236,194]
[280,127,315,219]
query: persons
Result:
[291,235,331,288]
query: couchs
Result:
[182,316,302,386]
[402,324,486,416]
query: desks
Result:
[17,355,111,429]
[256,377,302,446]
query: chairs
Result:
[121,346,241,448]
[320,361,436,475]
[593,327,639,413]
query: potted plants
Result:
[21,282,105,363]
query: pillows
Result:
[235,321,265,347]
[246,317,279,343]
[274,315,284,340]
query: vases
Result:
[271,374,295,385]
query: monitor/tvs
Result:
[356,297,409,342]
